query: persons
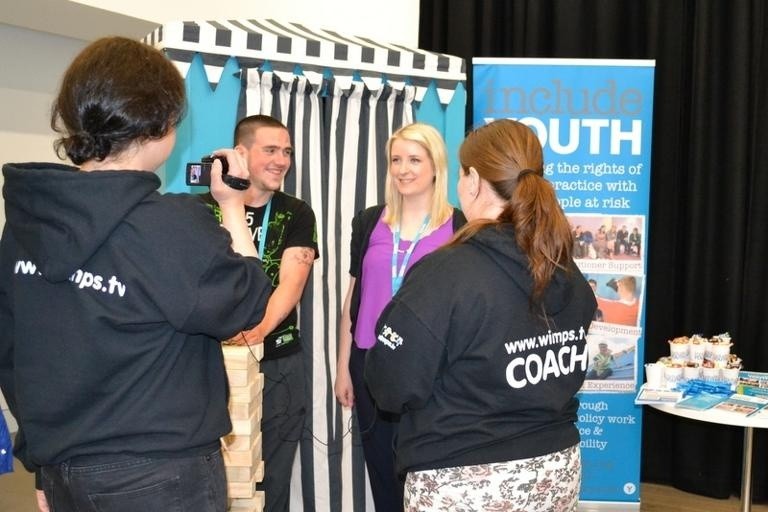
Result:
[0,34,276,511]
[584,343,622,380]
[333,121,465,510]
[588,279,604,323]
[596,275,639,327]
[568,218,643,259]
[363,117,597,512]
[190,170,197,180]
[193,114,321,510]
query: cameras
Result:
[186,153,229,186]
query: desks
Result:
[642,368,768,512]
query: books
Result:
[710,392,768,418]
[729,370,768,399]
[675,393,726,412]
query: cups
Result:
[643,337,743,390]
